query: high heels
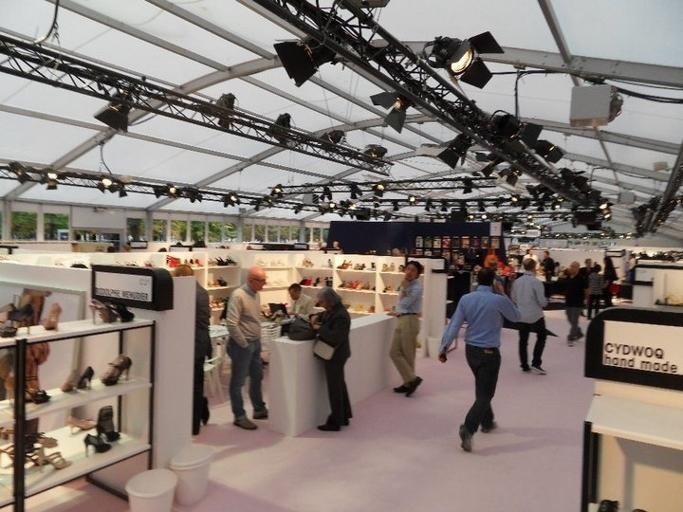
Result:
[24,385,52,404]
[45,304,60,330]
[5,303,36,336]
[62,370,79,393]
[77,368,94,391]
[102,357,132,384]
[83,433,113,457]
[96,422,121,442]
[104,300,135,322]
[68,415,99,432]
[90,300,118,323]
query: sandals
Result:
[1,445,15,458]
[167,256,180,267]
[0,428,6,440]
[26,450,48,466]
[327,257,334,268]
[337,259,352,269]
[38,447,72,473]
[23,432,33,452]
[37,435,56,445]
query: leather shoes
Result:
[344,420,351,425]
[406,376,423,396]
[399,265,402,270]
[202,397,211,425]
[338,279,376,291]
[184,258,203,267]
[393,384,410,393]
[317,423,342,431]
[382,263,395,273]
[344,302,376,314]
[299,276,333,289]
[209,254,237,266]
[303,258,312,265]
[598,499,616,511]
[460,424,473,454]
[481,421,498,432]
[209,278,228,287]
[383,284,393,293]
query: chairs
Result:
[203,340,226,404]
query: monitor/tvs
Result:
[268,303,288,319]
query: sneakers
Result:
[522,364,533,371]
[531,362,547,376]
[567,337,573,348]
[234,416,259,431]
[254,410,270,418]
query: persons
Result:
[226,266,271,431]
[311,287,354,432]
[587,263,609,319]
[539,250,555,284]
[521,248,540,271]
[510,257,549,374]
[172,264,213,436]
[579,259,600,314]
[286,282,314,320]
[624,253,638,281]
[389,258,426,399]
[436,266,522,453]
[600,257,617,307]
[562,260,590,342]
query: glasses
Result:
[253,278,267,283]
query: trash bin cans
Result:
[170,442,214,502]
[125,469,176,512]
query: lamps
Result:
[153,183,177,199]
[266,114,291,141]
[349,185,363,199]
[408,194,417,205]
[463,177,474,194]
[425,198,435,211]
[215,93,236,128]
[371,184,385,197]
[339,200,353,216]
[329,203,337,212]
[498,165,523,186]
[475,150,505,178]
[335,0,390,8]
[363,144,387,162]
[437,132,478,170]
[491,110,523,142]
[558,167,601,201]
[271,184,284,198]
[518,122,544,149]
[40,168,66,189]
[536,139,564,162]
[494,197,505,208]
[460,201,471,211]
[95,92,134,131]
[97,175,127,197]
[11,161,33,183]
[370,91,415,134]
[598,198,615,209]
[440,199,449,211]
[320,187,332,200]
[222,192,240,208]
[273,35,337,87]
[527,183,564,211]
[421,30,505,89]
[320,130,344,153]
[511,194,520,206]
[478,200,487,211]
[601,208,611,219]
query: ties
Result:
[293,301,297,313]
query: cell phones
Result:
[493,278,498,287]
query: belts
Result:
[396,313,420,317]
[466,344,498,353]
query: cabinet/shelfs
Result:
[0,320,155,512]
[0,264,87,393]
[630,262,683,308]
[332,253,374,316]
[580,309,683,512]
[294,252,332,307]
[375,256,447,361]
[206,251,254,323]
[7,252,95,270]
[163,253,206,288]
[96,252,161,270]
[254,251,293,306]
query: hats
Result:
[477,268,495,286]
[523,258,537,271]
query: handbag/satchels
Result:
[288,314,316,341]
[312,315,349,361]
